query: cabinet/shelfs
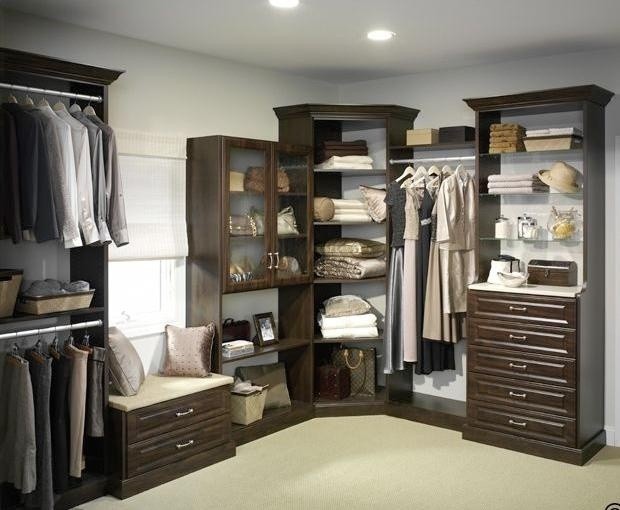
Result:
[107,372,234,502]
[385,141,475,432]
[272,102,421,418]
[185,135,314,447]
[461,84,614,467]
[0,45,128,510]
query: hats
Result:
[537,161,583,194]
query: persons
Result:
[261,317,275,341]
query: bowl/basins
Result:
[496,271,529,287]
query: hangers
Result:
[2,82,99,118]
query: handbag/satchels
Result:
[333,346,377,397]
[229,214,257,237]
[243,166,289,193]
[222,317,252,343]
[251,206,300,236]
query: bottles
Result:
[494,214,511,239]
[516,213,540,240]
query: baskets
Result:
[16,288,96,316]
[231,377,270,426]
[522,135,582,152]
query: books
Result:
[524,126,584,137]
[222,339,256,358]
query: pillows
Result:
[107,322,216,399]
